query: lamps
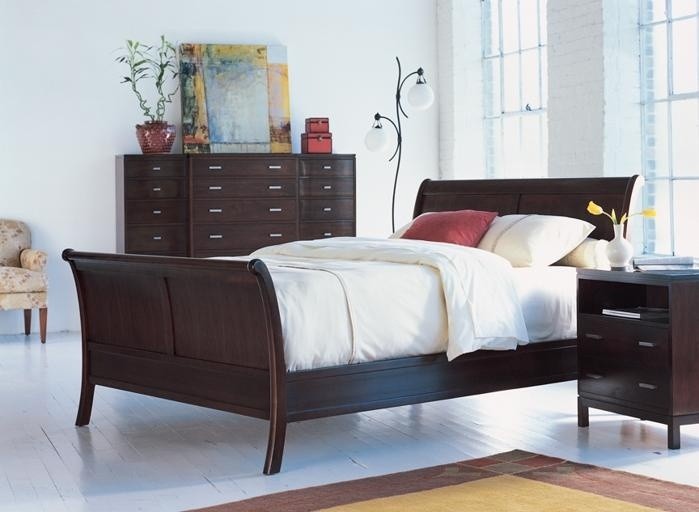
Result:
[364,56,435,235]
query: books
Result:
[633,256,698,274]
[603,307,668,320]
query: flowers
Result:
[589,200,654,225]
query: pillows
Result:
[399,210,497,248]
[476,214,598,266]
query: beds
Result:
[62,174,643,475]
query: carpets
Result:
[180,448,699,512]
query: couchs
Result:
[1,219,49,344]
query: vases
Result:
[604,225,634,267]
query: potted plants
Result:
[109,35,182,154]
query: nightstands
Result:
[575,269,699,452]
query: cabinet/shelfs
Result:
[123,153,356,256]
